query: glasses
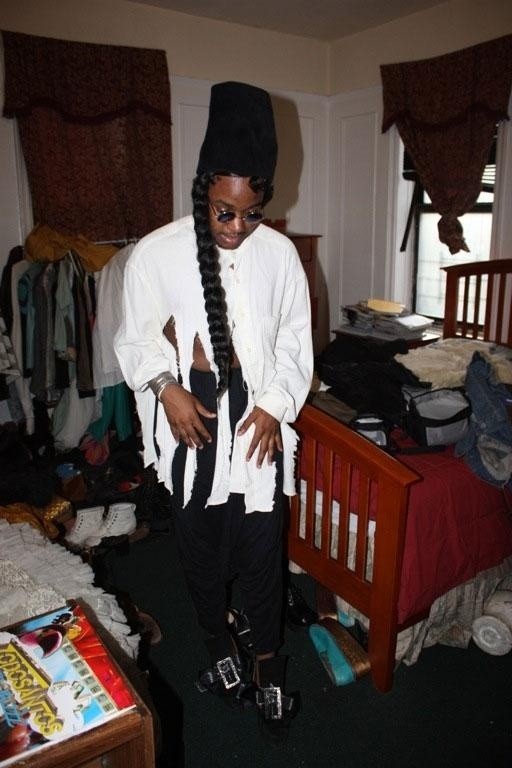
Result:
[205,197,270,224]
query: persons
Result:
[111,171,313,723]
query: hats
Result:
[194,78,277,179]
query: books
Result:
[341,296,435,337]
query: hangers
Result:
[93,236,139,249]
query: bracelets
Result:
[148,370,181,403]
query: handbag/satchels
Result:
[399,382,474,447]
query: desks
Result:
[288,233,322,330]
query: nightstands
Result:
[331,329,440,350]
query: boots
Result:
[65,506,105,546]
[86,503,139,547]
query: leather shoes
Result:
[284,583,319,633]
[206,624,248,710]
[250,656,298,751]
[307,617,371,690]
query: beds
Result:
[287,259,511,693]
